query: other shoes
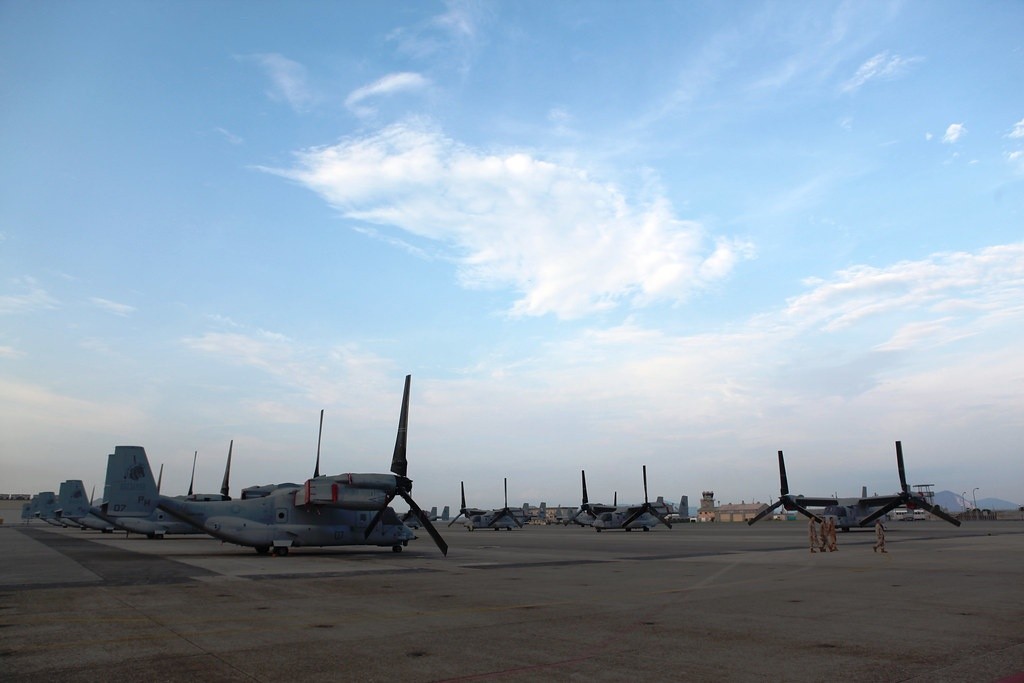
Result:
[811,547,839,553]
[873,546,877,552]
[881,550,887,553]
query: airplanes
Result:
[106,374,448,558]
[22,409,233,540]
[397,491,449,530]
[747,441,961,532]
[564,465,689,533]
[448,478,546,531]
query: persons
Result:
[820,517,838,552]
[808,517,826,553]
[873,519,888,553]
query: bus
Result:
[890,510,926,520]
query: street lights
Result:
[962,492,966,509]
[973,488,979,519]
